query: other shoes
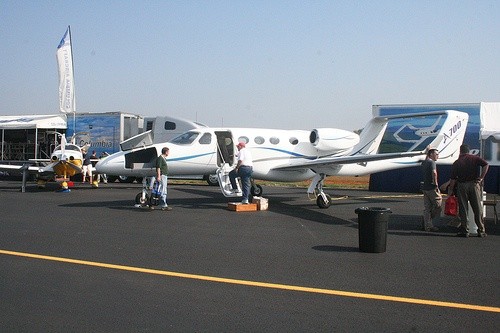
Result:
[459,232,468,237]
[478,233,487,238]
[430,226,439,231]
[149,206,154,209]
[242,199,248,203]
[230,189,239,193]
[161,207,171,210]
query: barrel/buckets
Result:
[467,191,487,234]
[355,207,392,253]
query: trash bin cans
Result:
[355,207,392,253]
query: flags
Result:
[56,29,73,114]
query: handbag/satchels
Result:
[444,196,458,216]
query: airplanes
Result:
[93,107,468,211]
[0,127,100,192]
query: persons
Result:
[229,141,253,203]
[90,151,109,183]
[155,147,172,210]
[421,146,443,232]
[82,149,92,184]
[449,145,489,237]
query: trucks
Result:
[364,100,500,194]
[0,110,206,183]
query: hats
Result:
[236,142,245,148]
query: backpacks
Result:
[151,181,161,197]
